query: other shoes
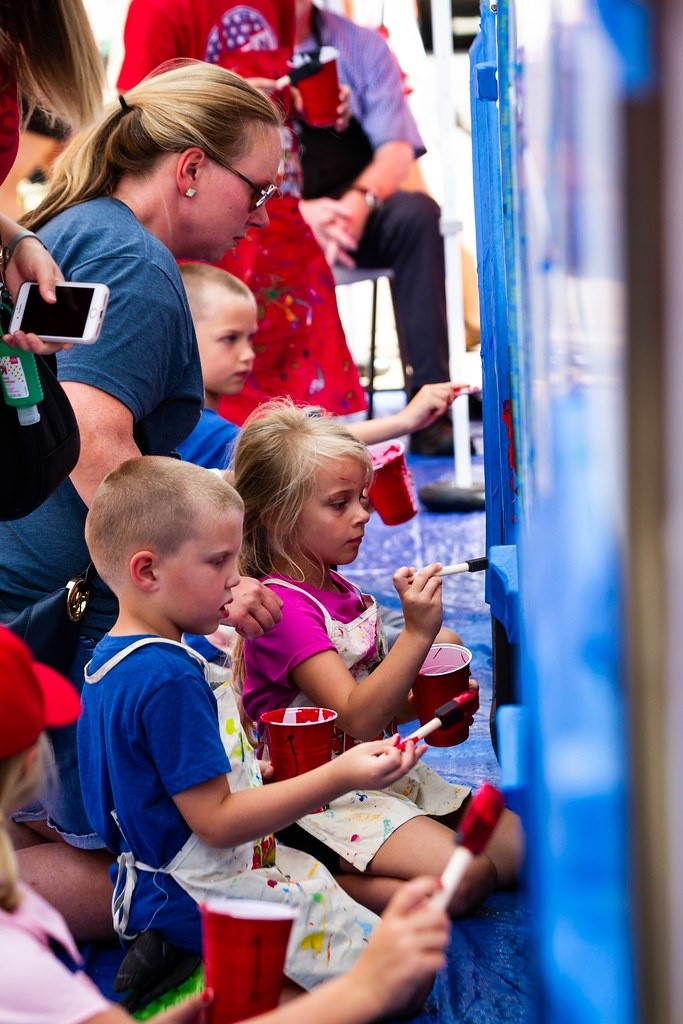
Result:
[411,426,475,454]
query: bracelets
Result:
[348,184,383,211]
[2,232,50,264]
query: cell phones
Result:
[8,280,110,345]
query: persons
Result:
[0,621,452,1024]
[0,0,528,1008]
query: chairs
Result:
[331,217,471,490]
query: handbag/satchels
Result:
[0,580,88,668]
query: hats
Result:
[0,300,81,524]
[0,626,81,754]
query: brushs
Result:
[429,779,504,909]
[407,557,488,583]
[397,687,478,749]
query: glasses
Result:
[211,157,276,209]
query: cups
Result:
[198,899,296,1024]
[365,441,420,526]
[260,707,338,814]
[287,46,340,126]
[411,643,472,746]
